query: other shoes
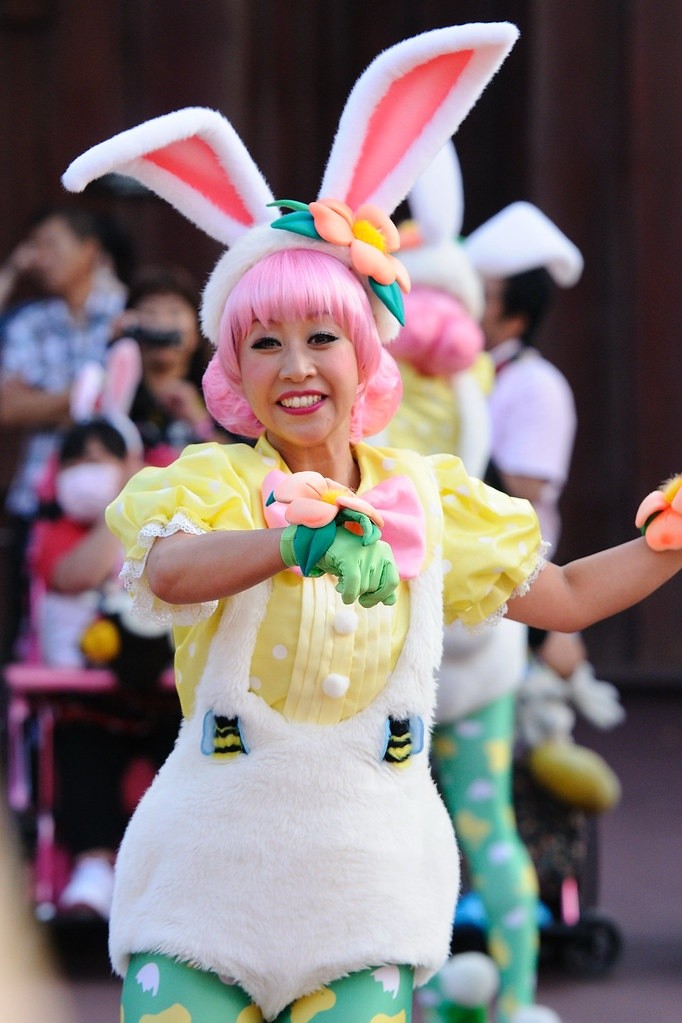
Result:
[59,853,113,924]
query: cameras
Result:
[126,326,185,349]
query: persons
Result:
[0,202,241,917]
[481,273,620,913]
[105,248,681,1023]
[386,252,557,1023]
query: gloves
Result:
[280,524,401,609]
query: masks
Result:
[55,458,118,519]
